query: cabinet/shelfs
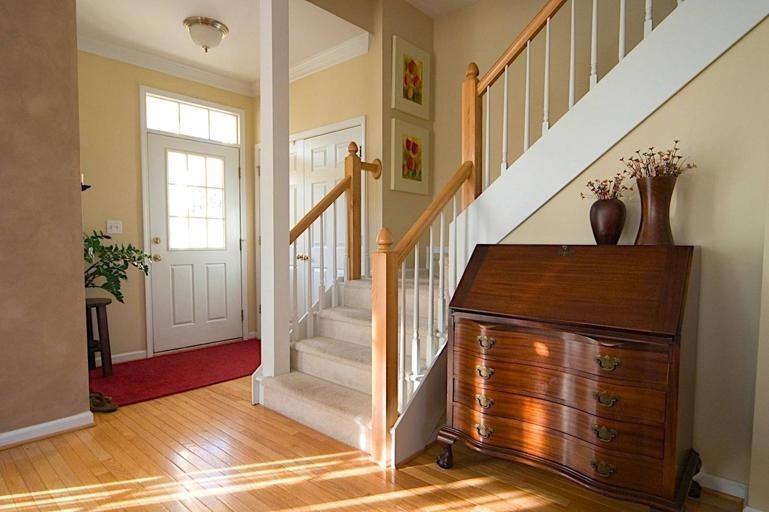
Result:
[435,244,703,512]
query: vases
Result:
[635,175,679,245]
[589,197,627,245]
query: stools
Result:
[84,298,112,376]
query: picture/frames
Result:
[391,35,432,196]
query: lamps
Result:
[182,15,230,54]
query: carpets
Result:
[88,336,262,413]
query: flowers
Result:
[579,138,697,201]
